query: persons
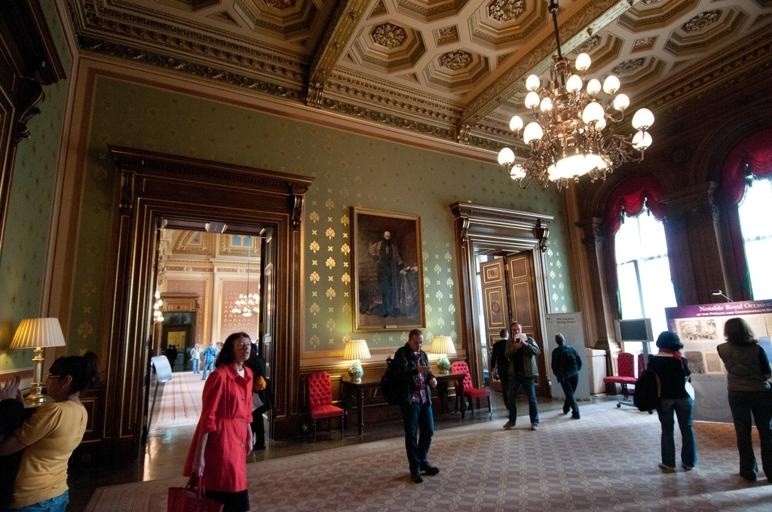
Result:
[181,330,257,510]
[167,339,265,384]
[489,326,515,413]
[247,344,273,454]
[550,332,583,420]
[366,229,406,320]
[502,319,544,432]
[714,316,770,483]
[647,331,697,471]
[0,347,98,510]
[393,330,441,482]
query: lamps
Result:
[710,288,734,302]
[343,337,373,383]
[9,310,66,404]
[494,3,654,191]
[432,332,457,375]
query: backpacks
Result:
[380,358,403,405]
[634,362,664,415]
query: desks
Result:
[343,372,470,434]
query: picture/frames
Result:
[352,207,426,332]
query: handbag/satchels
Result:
[167,474,224,511]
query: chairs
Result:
[617,354,654,415]
[303,370,344,443]
[601,349,634,397]
[452,362,492,416]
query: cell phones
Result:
[516,338,520,343]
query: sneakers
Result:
[420,465,439,475]
[504,423,515,429]
[410,473,424,483]
[531,424,540,432]
[681,462,694,470]
[658,462,677,470]
